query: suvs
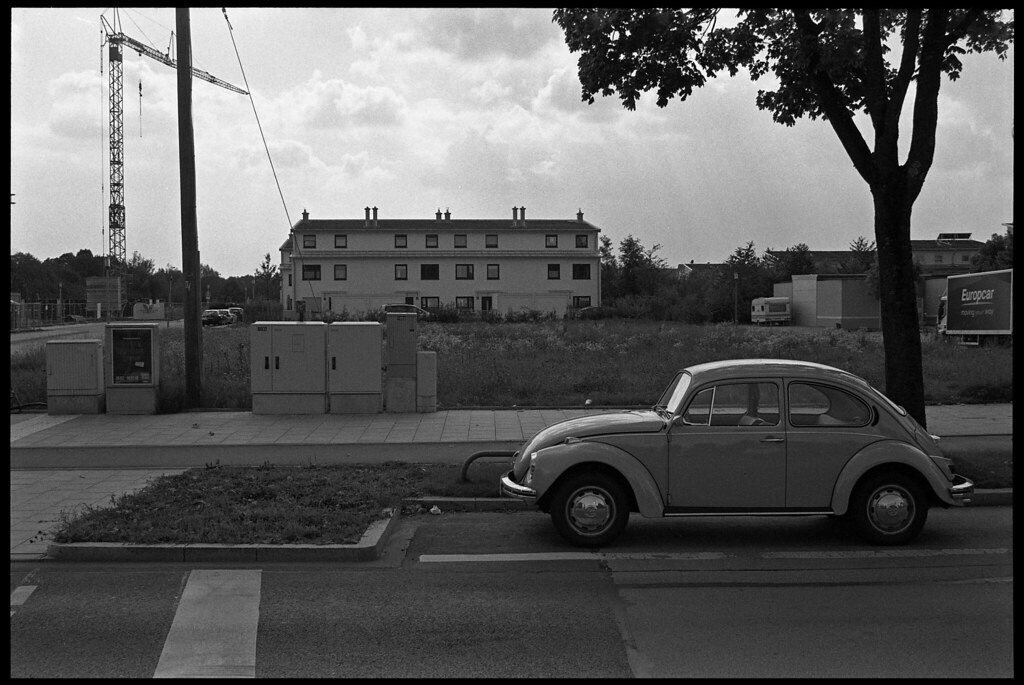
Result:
[229,308,243,321]
[375,304,438,324]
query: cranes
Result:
[100,8,249,318]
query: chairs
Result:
[758,422,777,426]
[818,414,843,424]
[738,409,765,426]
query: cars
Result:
[202,310,227,327]
[575,306,612,320]
[218,310,238,324]
[499,360,974,546]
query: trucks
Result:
[937,268,1013,345]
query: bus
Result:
[751,297,791,326]
